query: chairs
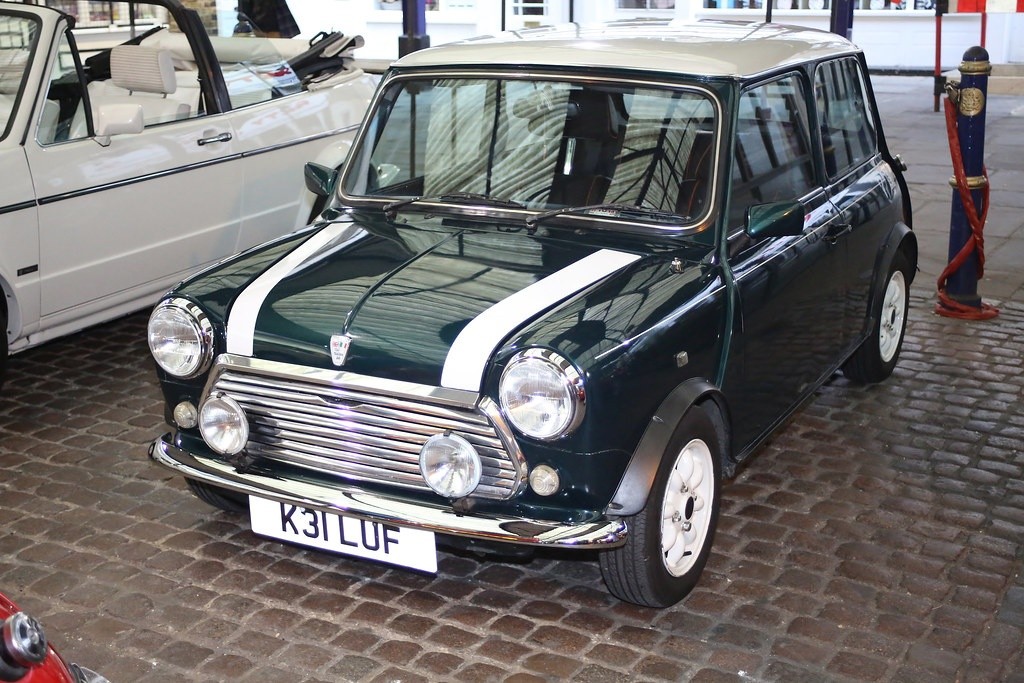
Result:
[67,46,190,140]
[0,50,62,145]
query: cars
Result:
[146,17,919,609]
[1,581,113,683]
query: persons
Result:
[237,0,301,40]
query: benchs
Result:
[142,29,318,73]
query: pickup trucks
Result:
[0,0,377,364]
